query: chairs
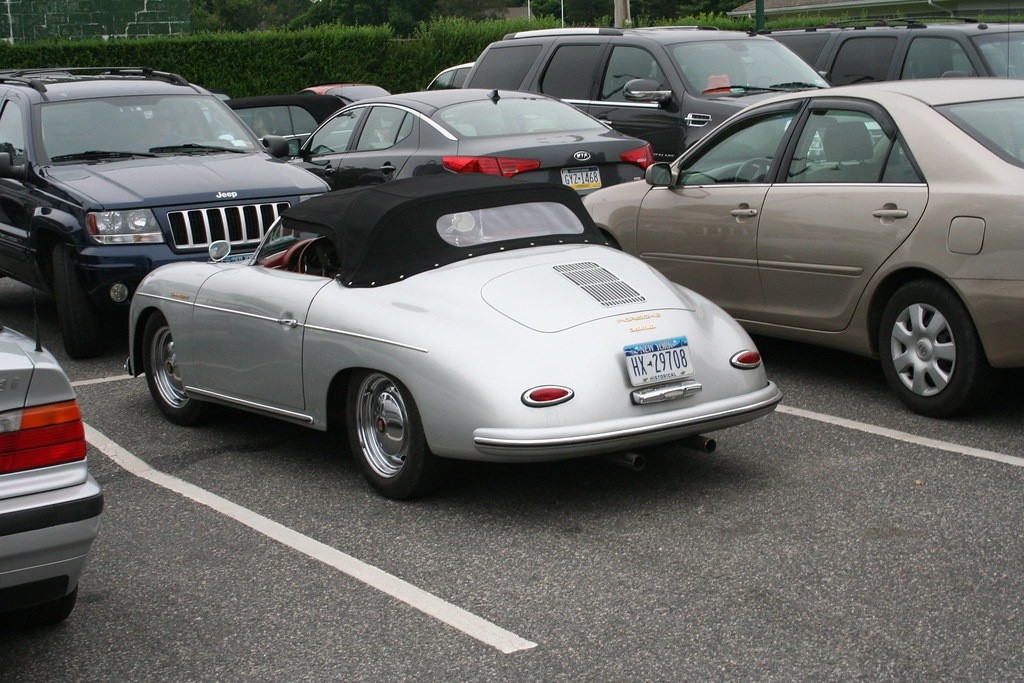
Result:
[804,120,883,185]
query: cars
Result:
[297,83,404,142]
[554,74,1024,371]
[283,87,657,208]
[220,94,362,165]
[421,60,478,91]
[0,324,104,640]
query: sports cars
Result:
[122,174,786,504]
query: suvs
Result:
[752,16,1024,89]
[0,63,336,362]
[461,22,892,177]
